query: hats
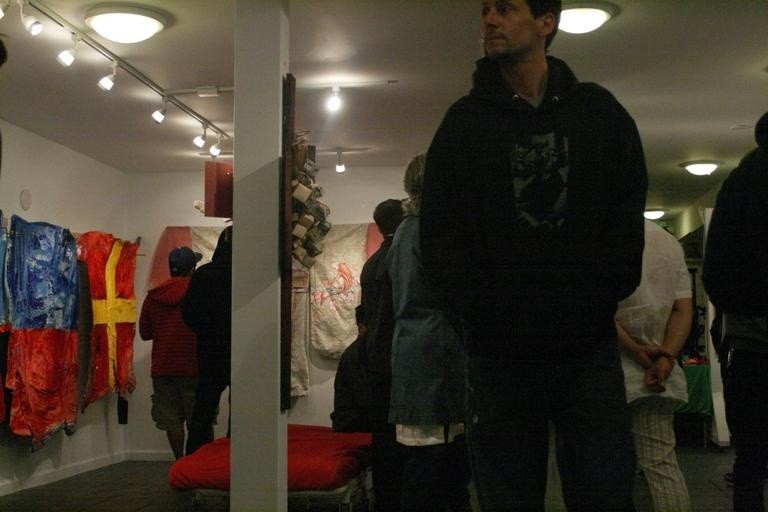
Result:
[169,246,203,267]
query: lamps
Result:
[0,0,230,158]
[560,1,620,34]
[297,78,392,111]
[85,6,166,44]
[644,209,668,220]
[677,160,723,176]
[199,148,370,174]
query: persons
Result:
[139,245,220,459]
[703,113,767,511]
[710,309,737,487]
[423,0,650,512]
[334,152,468,511]
[183,226,230,453]
[613,216,694,511]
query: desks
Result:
[676,364,713,449]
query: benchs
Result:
[169,424,372,512]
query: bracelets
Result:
[665,353,676,365]
